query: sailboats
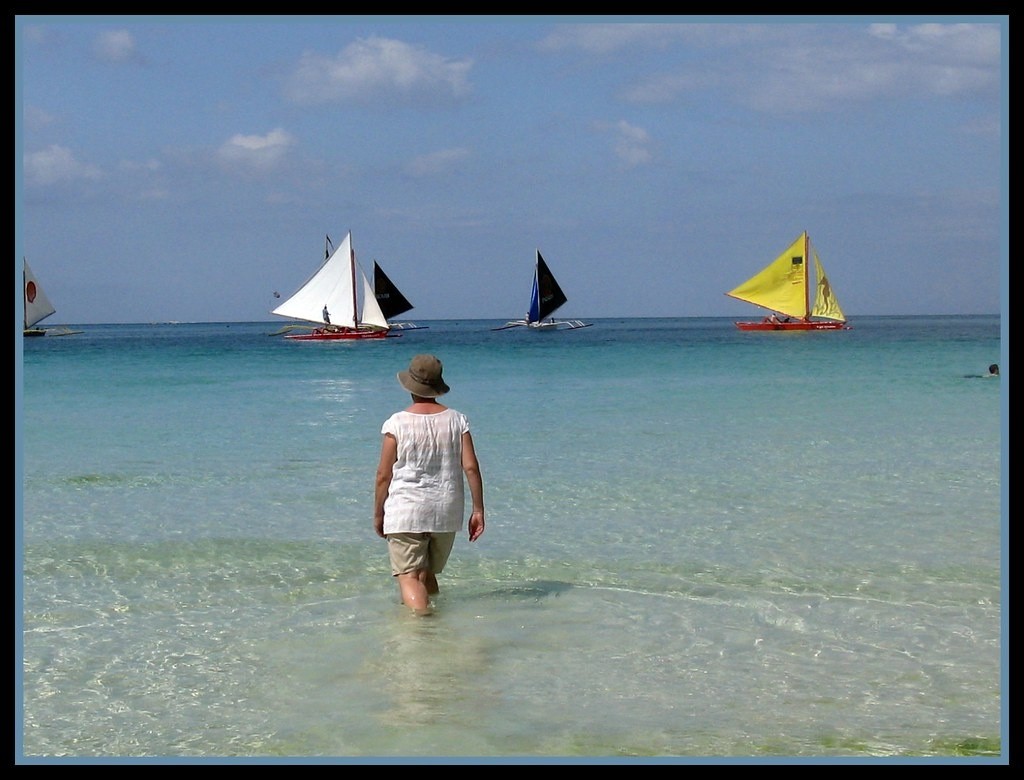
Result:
[723,229,850,331]
[492,247,595,332]
[21,254,57,337]
[269,228,429,340]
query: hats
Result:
[396,355,453,399]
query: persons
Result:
[989,364,1000,375]
[771,313,776,321]
[374,355,484,609]
[323,304,331,325]
[525,312,529,322]
